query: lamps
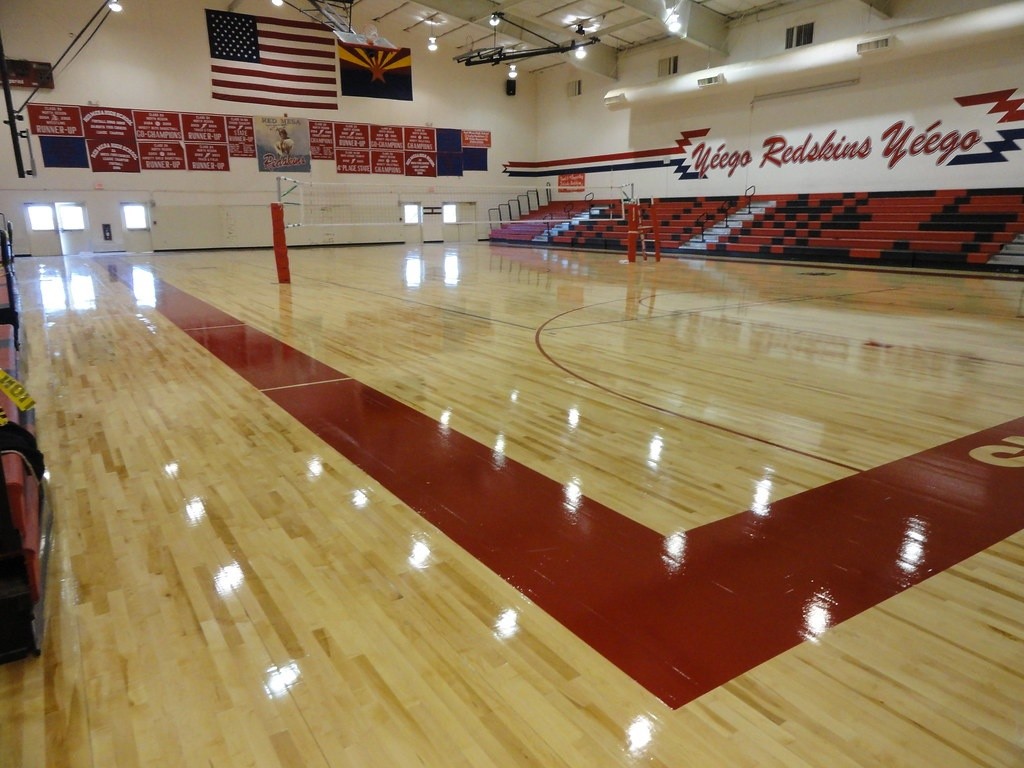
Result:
[508,48,518,78]
[428,18,437,52]
[489,12,501,26]
[106,0,122,13]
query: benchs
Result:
[490,196,1024,273]
